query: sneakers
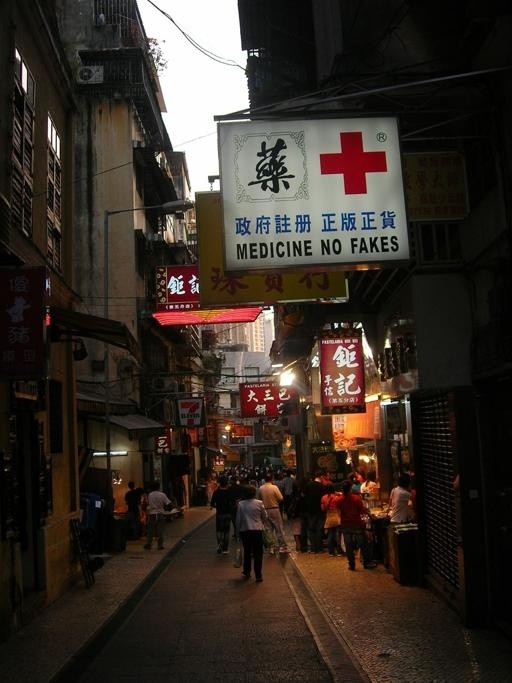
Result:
[216,543,230,555]
[269,547,292,555]
[241,571,263,582]
[309,550,344,557]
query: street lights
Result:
[99,198,197,558]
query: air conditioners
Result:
[152,376,175,392]
[74,65,106,87]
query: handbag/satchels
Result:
[262,526,276,548]
[233,548,242,568]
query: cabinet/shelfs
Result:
[366,512,421,588]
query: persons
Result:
[206,455,416,557]
[123,481,145,541]
[330,478,377,568]
[235,486,268,581]
[142,482,175,550]
